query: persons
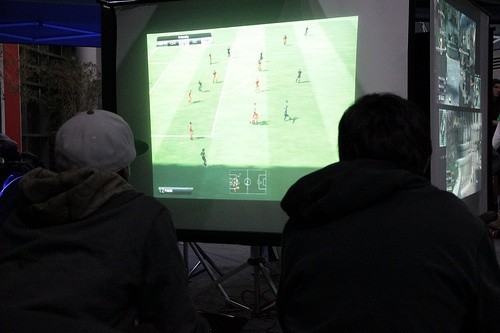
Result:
[0,109,212,333]
[277,92,500,333]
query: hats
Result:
[55,110,149,173]
